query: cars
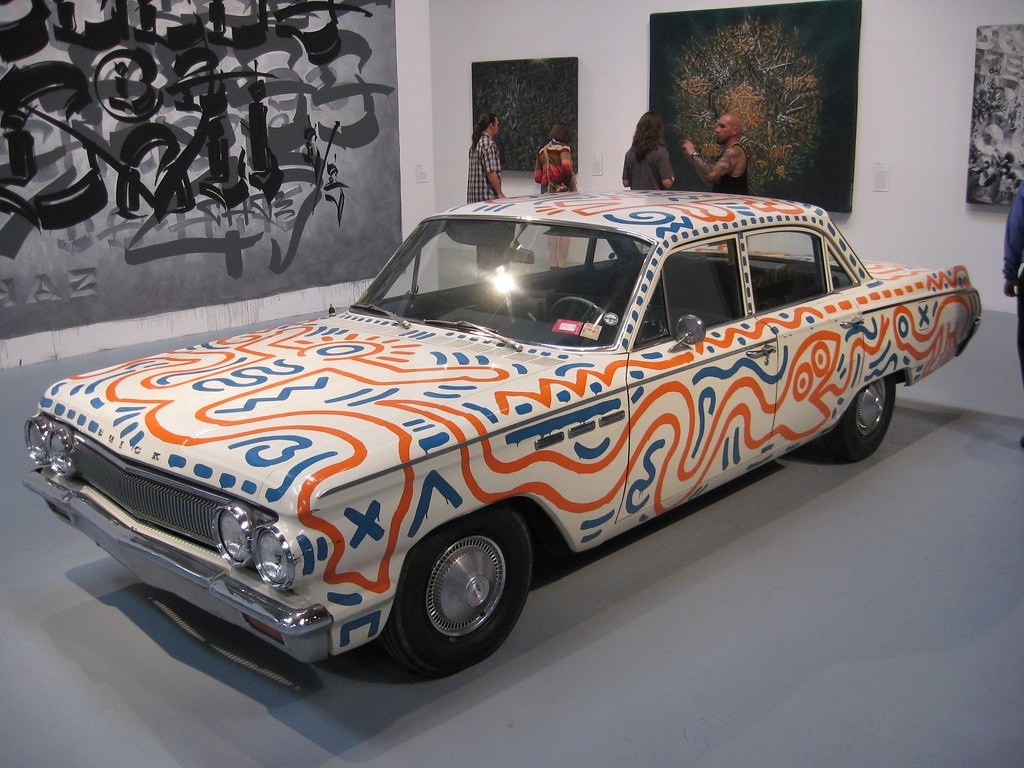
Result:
[18,189,983,681]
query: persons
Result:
[466,114,511,279]
[534,122,578,270]
[1002,179,1024,448]
[622,111,674,191]
[683,113,749,194]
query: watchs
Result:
[691,151,700,159]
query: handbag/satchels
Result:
[548,178,578,193]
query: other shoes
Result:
[550,264,564,271]
